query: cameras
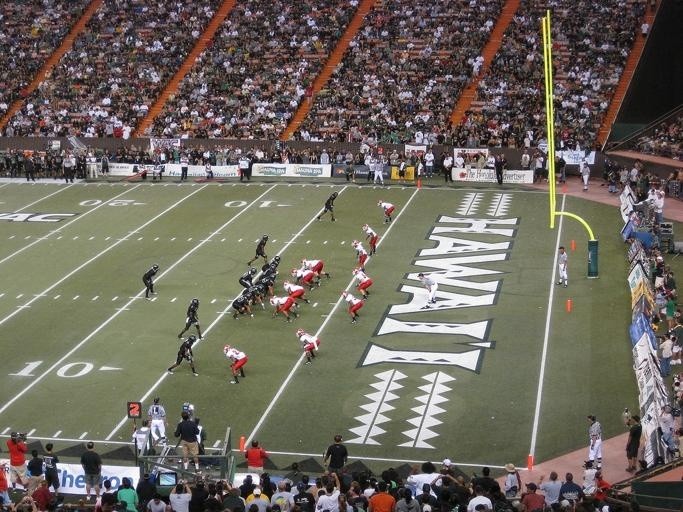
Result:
[672,374,681,386]
[649,257,657,261]
[179,479,184,484]
[5,467,9,471]
[655,335,664,341]
[624,408,631,425]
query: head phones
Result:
[334,435,343,442]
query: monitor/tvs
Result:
[158,472,177,486]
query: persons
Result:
[318,190,338,222]
[0,0,656,184]
[178,298,206,341]
[377,200,395,224]
[0,396,621,512]
[167,335,200,377]
[342,224,378,323]
[295,327,320,365]
[232,235,332,324]
[142,264,160,300]
[223,345,249,384]
[579,112,683,473]
[417,273,439,309]
[556,247,568,288]
